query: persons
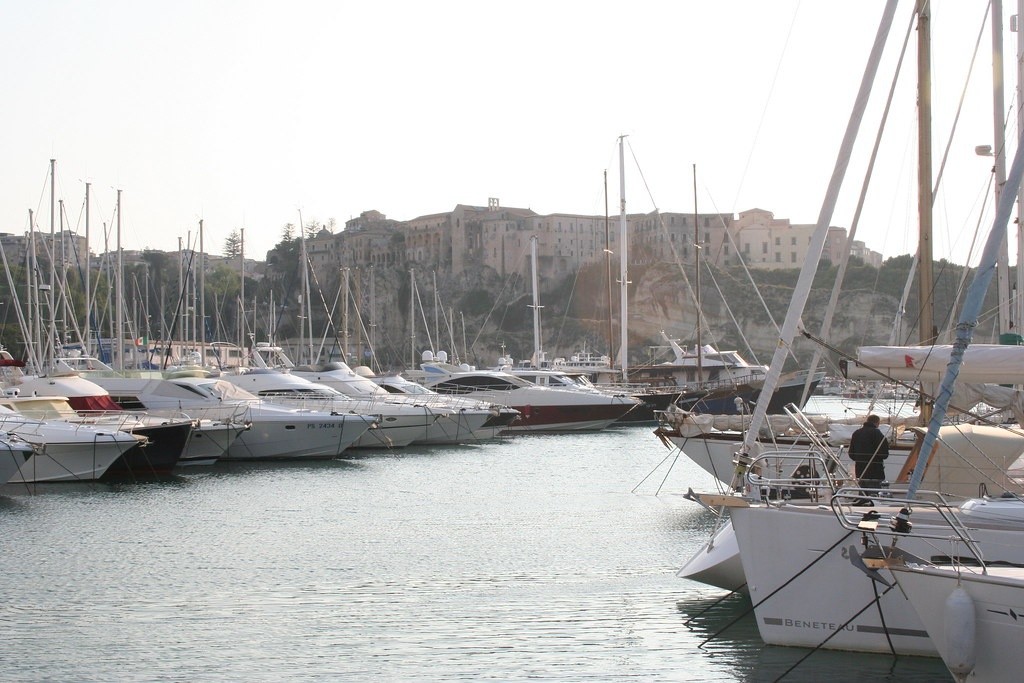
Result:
[848,415,889,507]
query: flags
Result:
[135,335,147,346]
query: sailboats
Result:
[0,133,829,489]
[632,1,1024,683]
